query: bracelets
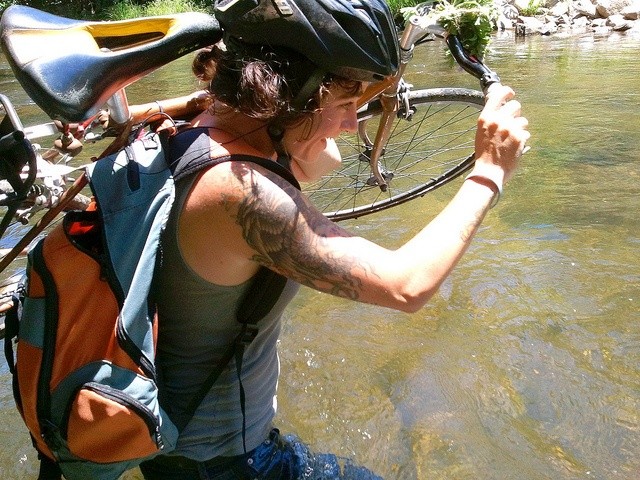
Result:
[466,159,505,208]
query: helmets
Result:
[212,0,401,84]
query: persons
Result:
[138,0,530,480]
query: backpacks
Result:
[4,106,300,478]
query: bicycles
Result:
[0,0,530,323]
